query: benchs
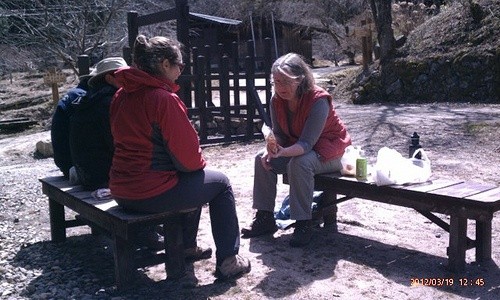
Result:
[283,165,500,270]
[38,175,181,281]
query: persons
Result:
[51,67,107,179]
[66,56,166,253]
[108,34,251,280]
[241,52,351,247]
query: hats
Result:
[78,65,97,81]
[87,57,128,87]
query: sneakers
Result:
[240,212,276,238]
[216,255,251,278]
[289,220,315,247]
[180,245,212,261]
[141,229,165,249]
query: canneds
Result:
[356,157,368,180]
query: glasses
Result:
[271,78,293,88]
[170,60,186,72]
